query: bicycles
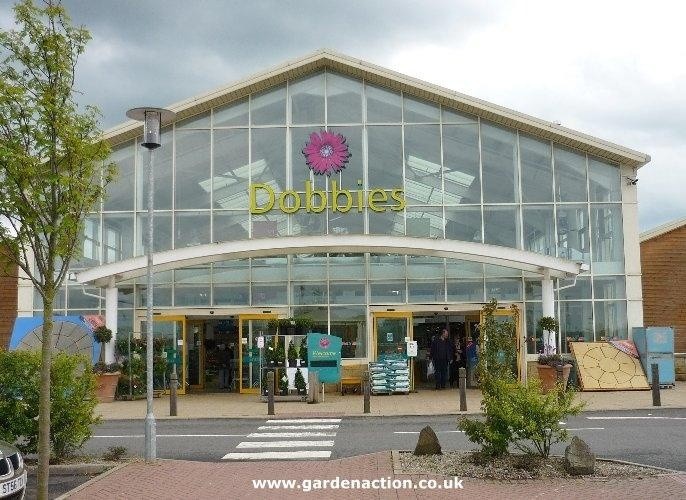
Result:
[165,374,190,393]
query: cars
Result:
[0,440,28,500]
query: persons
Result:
[216,342,234,388]
[428,328,476,389]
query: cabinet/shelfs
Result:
[259,330,314,403]
[116,331,165,402]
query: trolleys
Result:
[227,359,250,392]
[339,364,364,396]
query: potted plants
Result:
[536,316,574,395]
[117,336,164,396]
[262,314,315,395]
[82,324,122,403]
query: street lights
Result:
[126,107,179,460]
